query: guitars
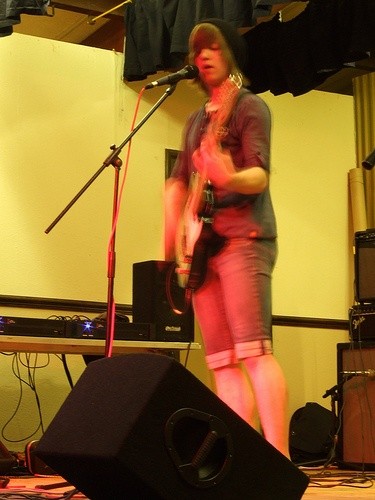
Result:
[174,73,242,291]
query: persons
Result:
[161,17,290,459]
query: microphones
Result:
[339,369,375,377]
[145,64,199,90]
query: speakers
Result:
[33,351,310,500]
[132,260,194,342]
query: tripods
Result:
[295,374,366,471]
[35,83,177,500]
